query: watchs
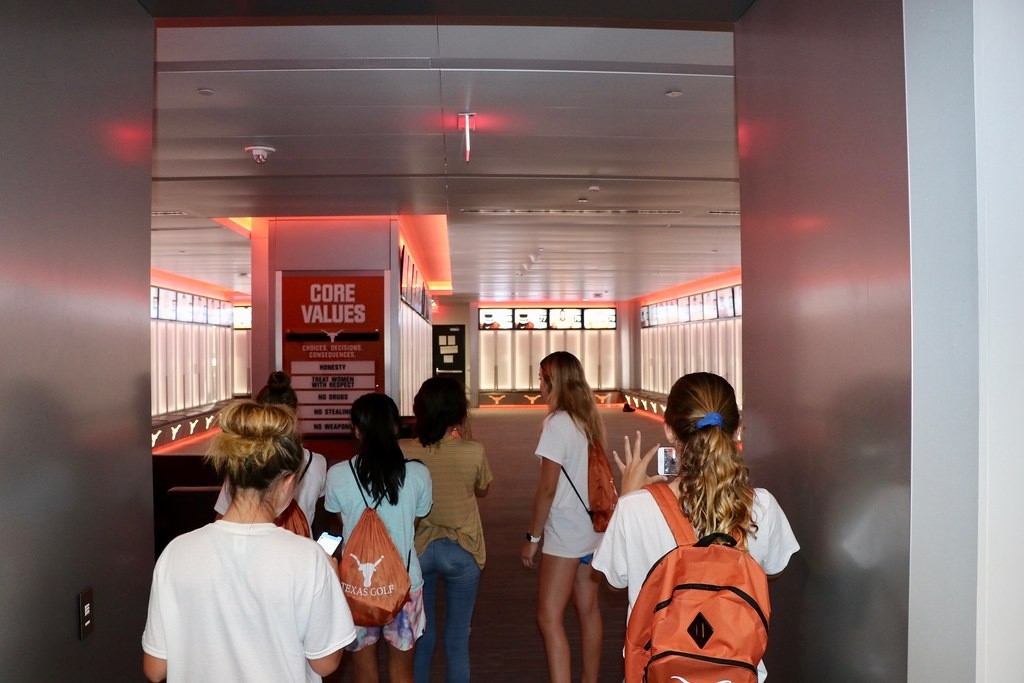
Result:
[525,531,542,543]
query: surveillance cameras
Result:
[252,150,267,164]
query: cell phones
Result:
[317,531,344,558]
[657,447,680,476]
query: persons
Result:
[141,399,359,683]
[521,350,607,683]
[396,374,493,683]
[323,393,433,683]
[592,371,800,683]
[213,371,328,544]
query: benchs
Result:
[475,387,669,416]
[152,398,249,447]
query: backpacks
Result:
[272,448,312,538]
[559,428,618,532]
[624,481,770,683]
[340,457,412,625]
